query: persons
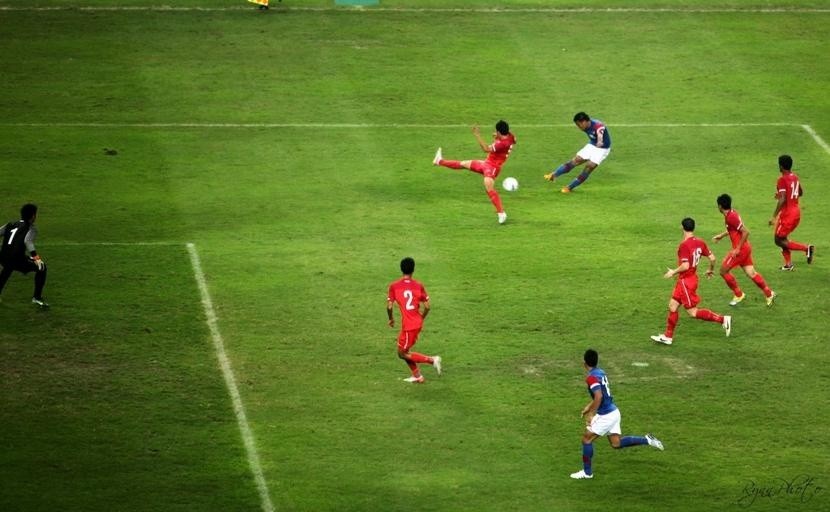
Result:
[649,217,733,346]
[768,154,814,272]
[1,203,48,308]
[432,121,517,223]
[386,257,443,384]
[545,112,612,194]
[568,350,665,481]
[710,193,778,309]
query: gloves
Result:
[33,259,46,271]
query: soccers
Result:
[503,177,518,191]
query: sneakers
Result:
[570,470,594,479]
[645,433,664,451]
[432,147,443,166]
[32,298,49,309]
[432,356,441,376]
[650,245,817,345]
[561,187,570,193]
[543,174,555,182]
[404,376,424,383]
[497,211,507,225]
